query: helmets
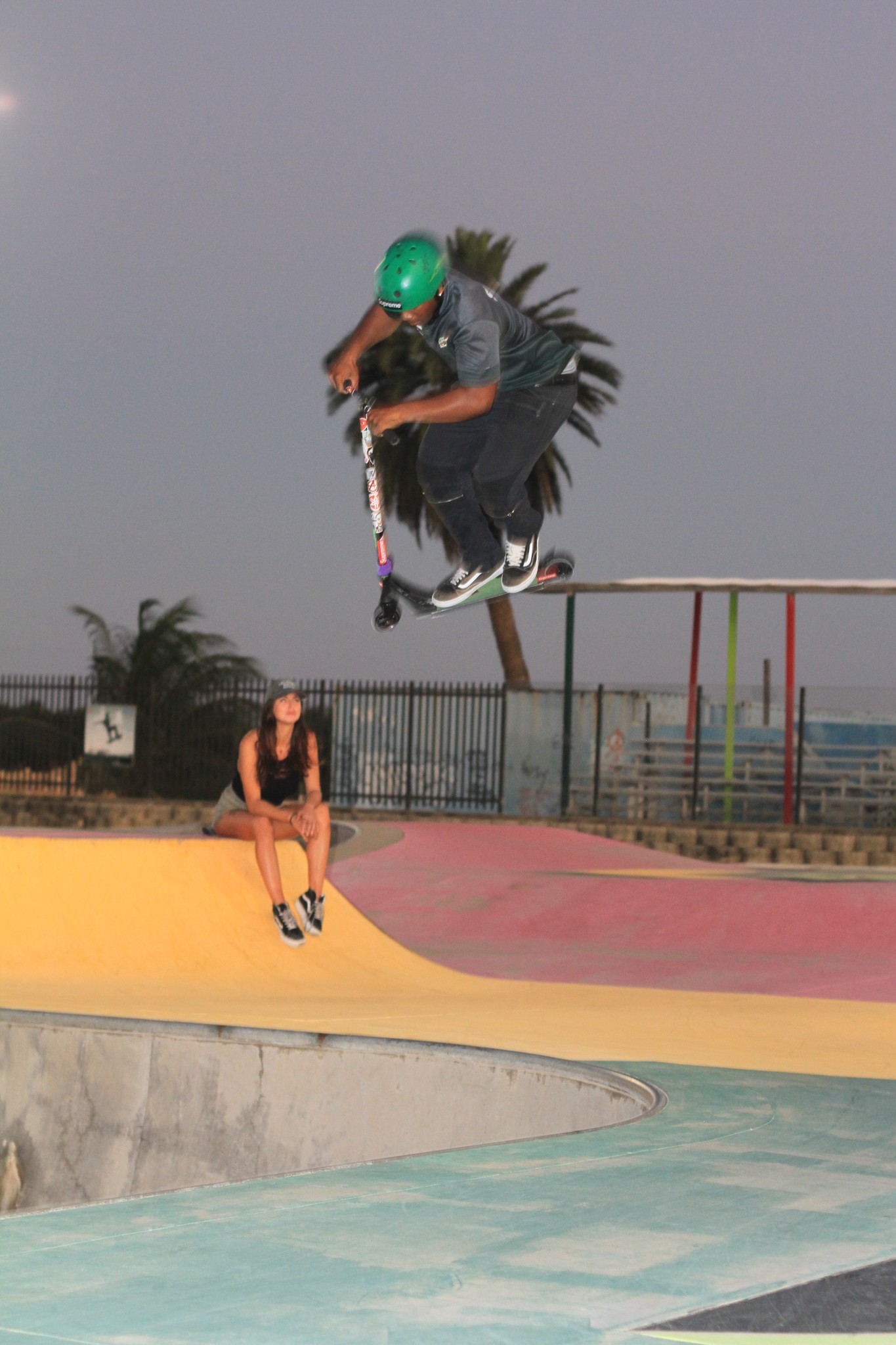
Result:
[373,238,445,313]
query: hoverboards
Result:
[342,378,577,632]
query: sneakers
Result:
[295,887,325,936]
[271,902,307,947]
[430,554,504,608]
[500,512,544,594]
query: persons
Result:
[326,237,579,611]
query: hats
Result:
[266,678,308,704]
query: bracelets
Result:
[290,812,302,826]
[212,679,330,946]
